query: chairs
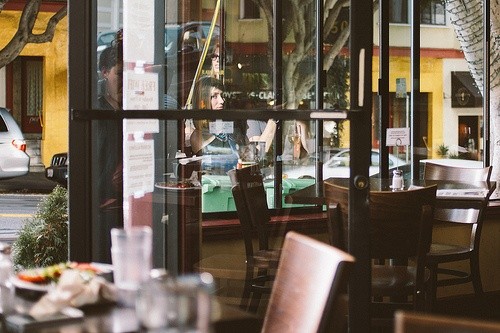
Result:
[226,162,500,333]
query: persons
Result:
[191,77,239,156]
[211,37,220,75]
[93,28,179,209]
[241,97,268,150]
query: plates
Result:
[155,181,203,190]
[12,262,113,292]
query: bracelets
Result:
[214,133,217,137]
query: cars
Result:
[262,147,414,191]
[1,106,31,180]
[202,142,243,174]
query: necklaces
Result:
[107,93,120,104]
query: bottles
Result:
[392,170,404,191]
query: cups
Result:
[110,226,152,290]
[149,268,214,333]
[0,254,15,314]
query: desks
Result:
[1,288,262,333]
[285,176,500,303]
